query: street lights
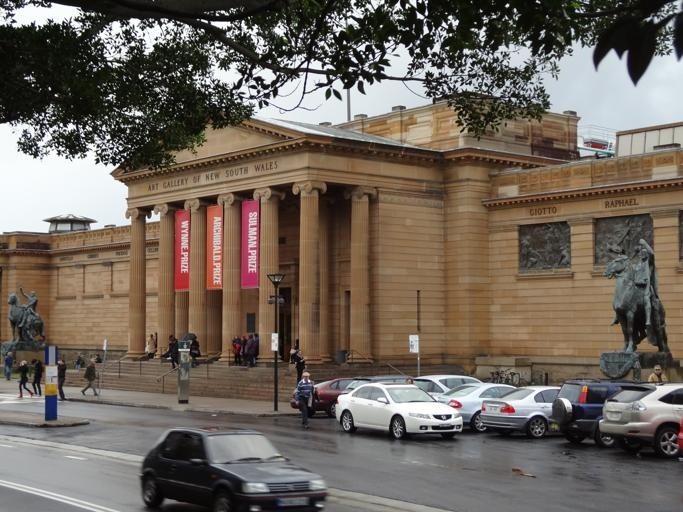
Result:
[266,272,286,412]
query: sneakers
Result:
[304,424,309,429]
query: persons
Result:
[231,334,260,368]
[597,215,653,265]
[3,348,104,398]
[608,237,656,331]
[146,332,156,360]
[292,371,321,429]
[288,338,299,365]
[166,333,178,370]
[188,336,201,368]
[647,364,669,383]
[17,286,38,329]
[293,350,306,389]
[519,225,569,270]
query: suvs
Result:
[139,426,328,510]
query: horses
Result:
[602,254,671,353]
[7,294,46,344]
